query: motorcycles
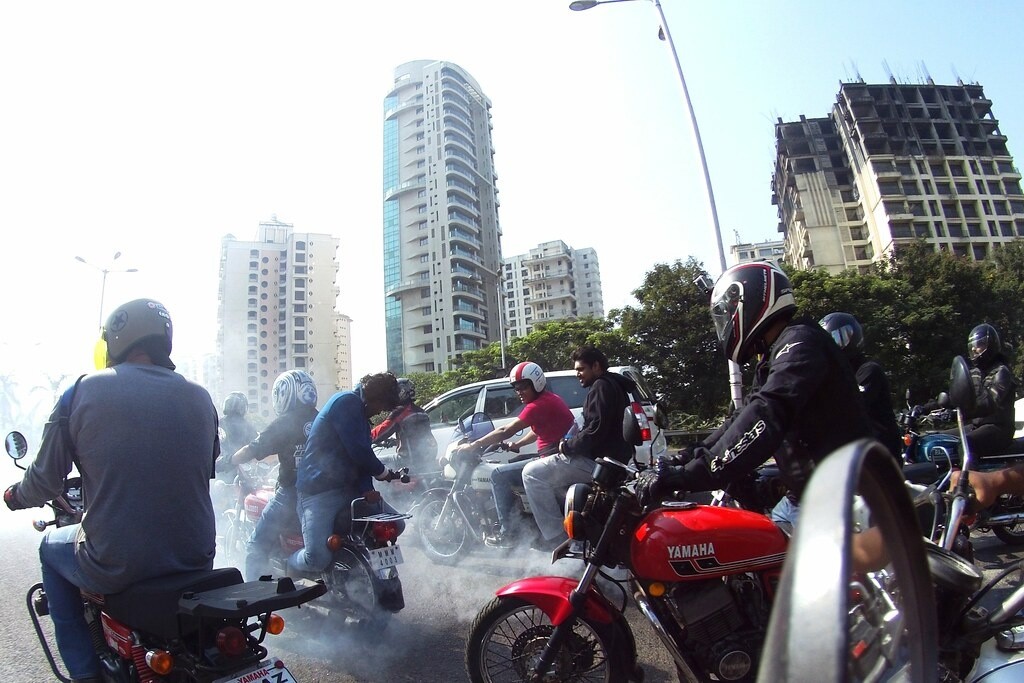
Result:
[1,428,329,683]
[212,456,409,636]
[417,411,581,567]
[463,353,1024,683]
[367,435,452,522]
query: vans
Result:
[373,365,671,520]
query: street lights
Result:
[76,249,139,334]
[570,0,729,281]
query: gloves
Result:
[635,469,674,510]
[215,452,238,473]
[4,484,26,510]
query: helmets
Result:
[272,369,318,415]
[711,261,795,363]
[101,297,172,359]
[509,361,547,393]
[397,377,416,403]
[969,323,1001,367]
[819,312,864,352]
[223,392,247,416]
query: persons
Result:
[4,299,221,683]
[218,392,259,483]
[522,347,635,552]
[817,313,905,469]
[371,378,439,493]
[633,260,872,528]
[913,324,1016,470]
[285,373,405,577]
[215,369,320,580]
[457,362,578,548]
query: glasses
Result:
[512,381,530,393]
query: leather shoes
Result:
[484,534,514,549]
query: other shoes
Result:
[532,533,568,552]
